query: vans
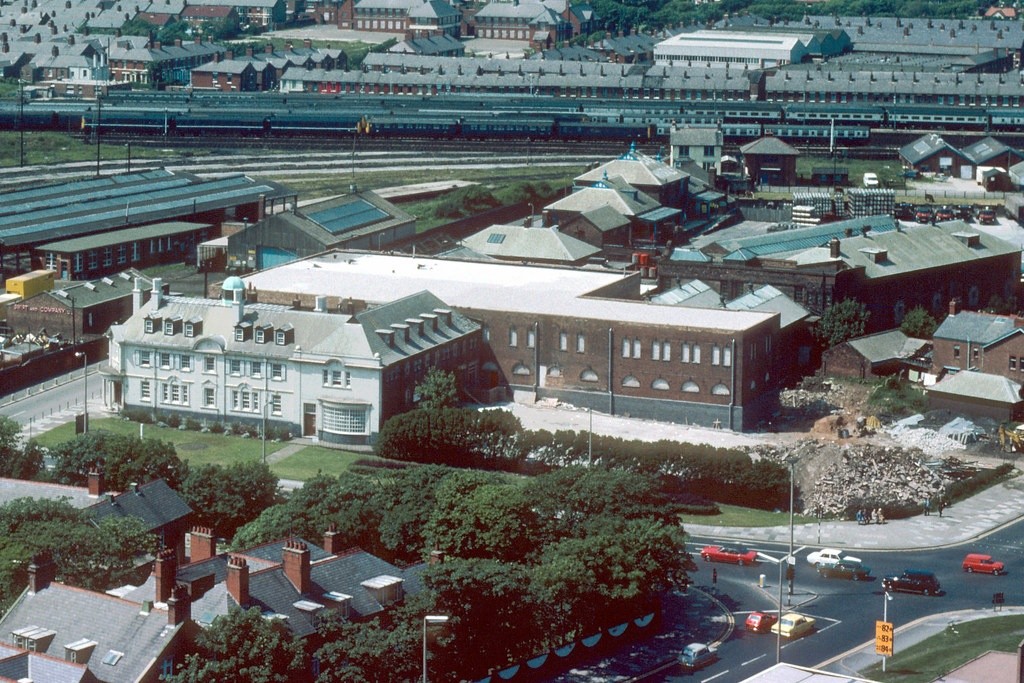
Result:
[963,554,1006,576]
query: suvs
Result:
[863,172,879,189]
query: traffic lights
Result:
[786,568,795,579]
[357,116,372,135]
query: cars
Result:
[771,612,816,639]
[678,642,719,670]
[701,544,758,566]
[744,612,778,632]
[934,173,953,182]
[806,549,862,569]
[897,203,996,224]
[881,569,942,596]
[817,560,872,581]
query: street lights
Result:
[782,452,797,593]
[424,616,449,682]
[75,352,88,434]
[564,174,569,198]
[528,203,534,226]
[585,408,591,463]
[57,290,75,360]
[263,401,275,466]
[883,592,894,671]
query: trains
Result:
[1,90,1024,143]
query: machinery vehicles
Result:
[998,423,1024,453]
